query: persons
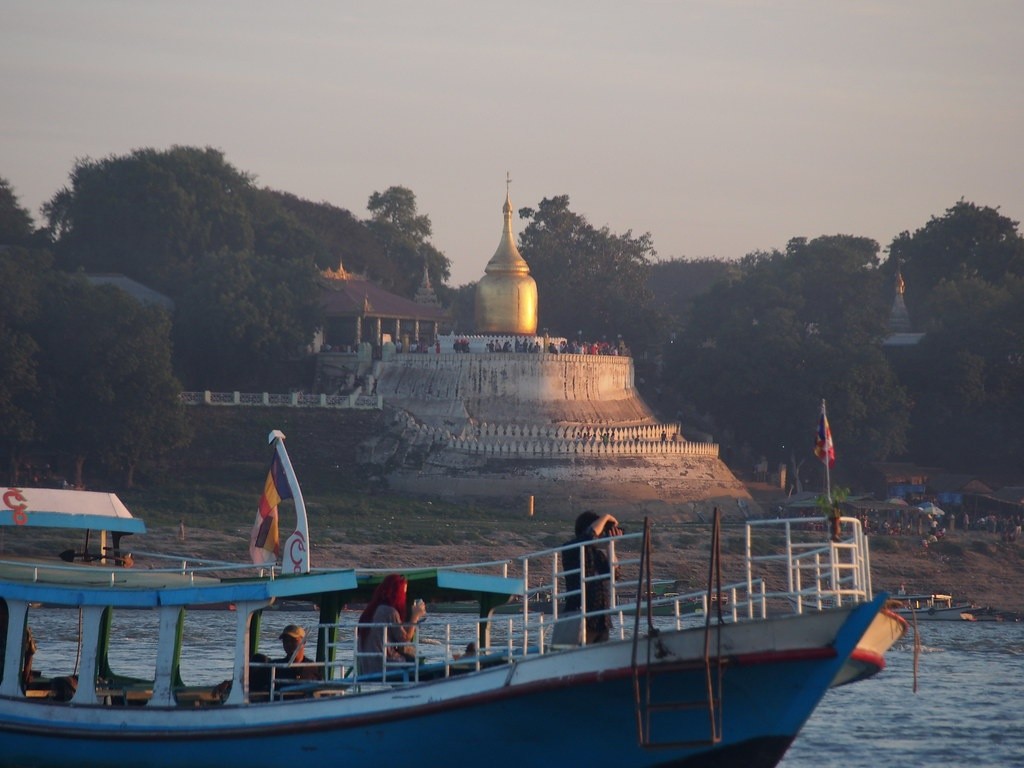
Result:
[316,330,636,357]
[561,509,624,645]
[768,501,1023,558]
[266,623,325,701]
[355,572,428,681]
[249,447,294,571]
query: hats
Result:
[278,625,305,638]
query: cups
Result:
[414,598,425,621]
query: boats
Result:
[278,567,729,618]
[784,591,1006,622]
[0,485,910,768]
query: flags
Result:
[813,409,837,470]
